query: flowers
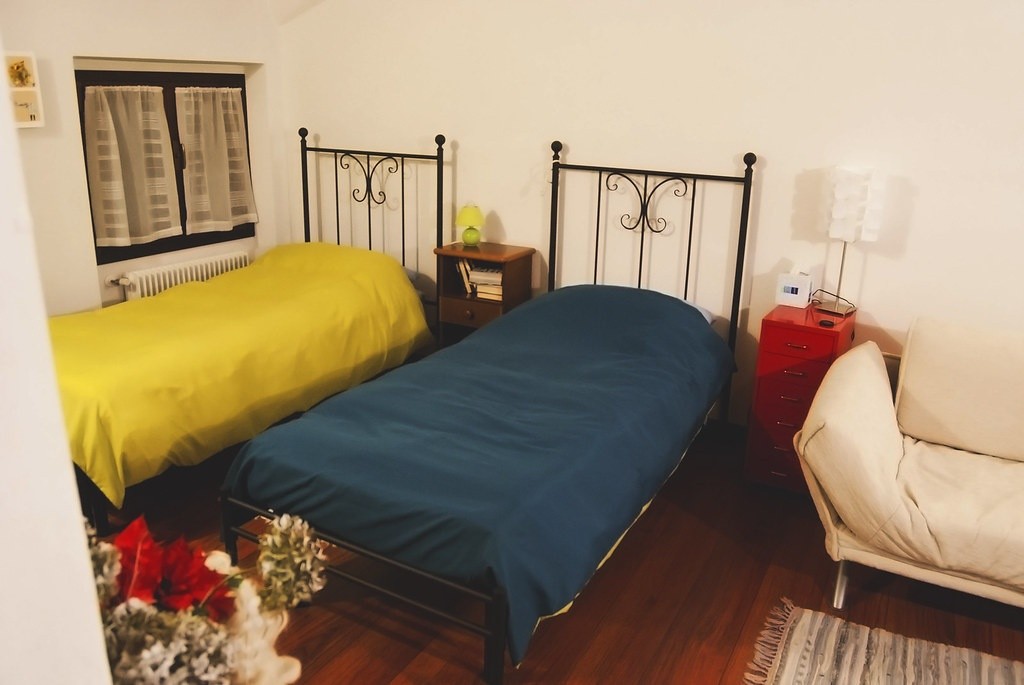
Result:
[89,511,331,685]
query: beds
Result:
[217,137,757,684]
[49,127,447,537]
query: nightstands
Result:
[432,241,535,348]
[741,304,856,498]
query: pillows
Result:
[681,299,715,326]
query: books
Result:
[462,257,480,289]
[452,256,467,295]
[477,292,503,302]
[457,257,475,293]
[468,262,504,286]
[476,284,503,296]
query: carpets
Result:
[743,586,1023,685]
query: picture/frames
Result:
[5,52,44,129]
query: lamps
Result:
[454,204,487,248]
[816,169,880,315]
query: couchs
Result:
[791,315,1024,612]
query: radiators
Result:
[126,249,249,301]
